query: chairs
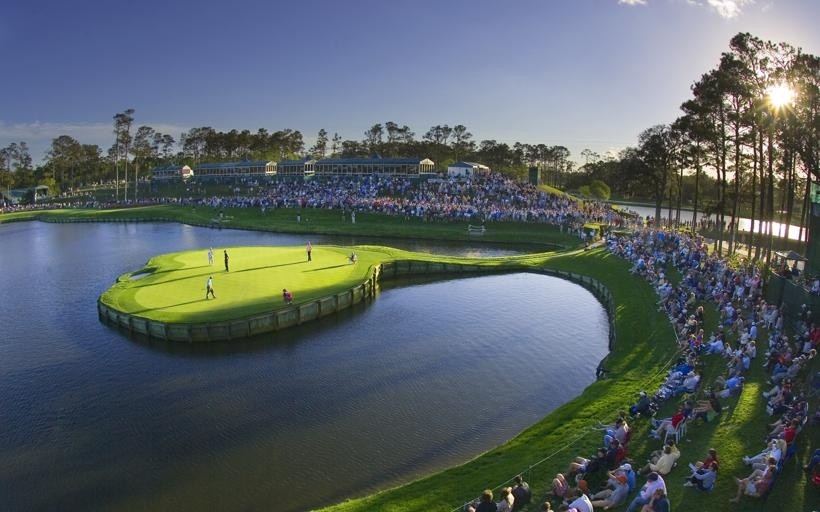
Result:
[664,415,688,444]
[745,402,808,505]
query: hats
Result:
[676,346,820,489]
[604,390,673,480]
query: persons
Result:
[346,251,358,264]
[281,290,295,304]
[204,274,218,300]
[207,246,213,266]
[0,167,655,226]
[306,240,314,261]
[462,221,815,512]
[223,249,231,272]
